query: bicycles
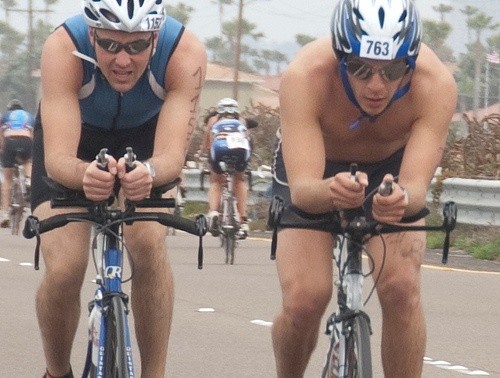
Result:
[9,147,25,236]
[219,161,245,265]
[21,146,207,378]
[266,163,458,377]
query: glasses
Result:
[343,53,408,80]
[94,28,153,55]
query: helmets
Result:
[215,97,238,114]
[7,99,21,108]
[330,0,422,61]
[82,0,166,31]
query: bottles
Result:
[90,290,103,366]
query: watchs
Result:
[141,161,156,177]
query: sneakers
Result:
[209,211,220,236]
[25,177,31,201]
[0,214,11,227]
[237,223,248,239]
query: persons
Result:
[267,0,458,378]
[0,99,35,228]
[206,98,254,239]
[31,0,207,378]
